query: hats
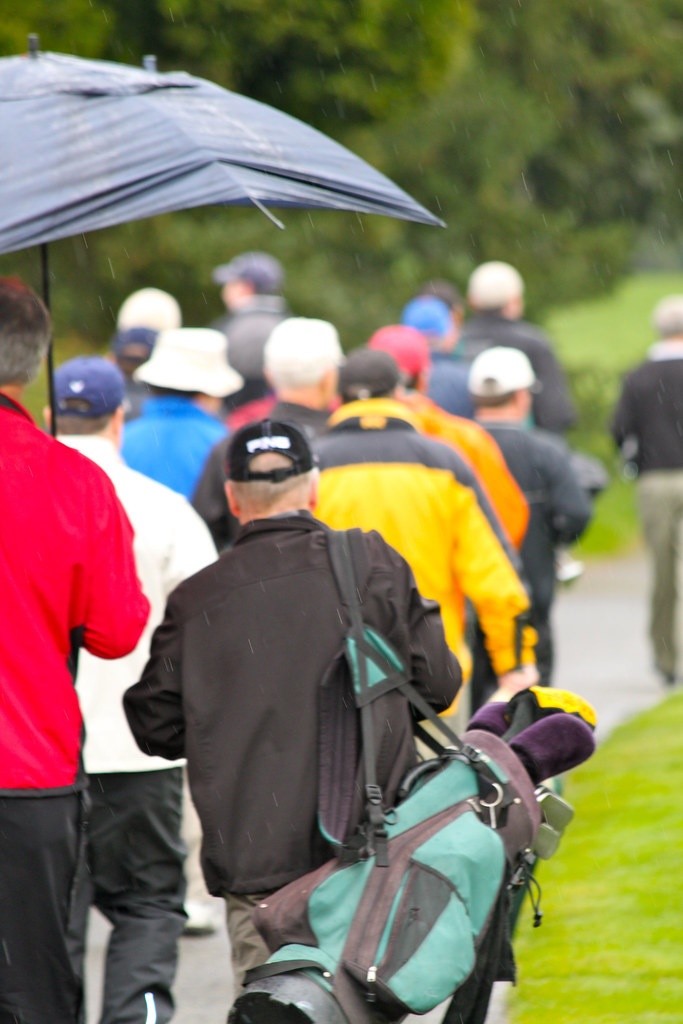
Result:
[262,317,346,387]
[53,356,132,417]
[224,419,319,482]
[402,296,453,338]
[112,287,182,365]
[213,250,283,294]
[370,324,430,377]
[467,345,536,396]
[133,326,244,398]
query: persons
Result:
[467,346,591,687]
[401,262,574,432]
[311,346,539,761]
[122,418,462,997]
[120,326,244,502]
[106,287,181,420]
[610,296,683,685]
[208,250,289,410]
[370,322,530,550]
[0,276,151,1024]
[43,356,219,1024]
[194,316,346,551]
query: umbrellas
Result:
[0,33,448,428]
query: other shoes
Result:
[181,902,216,932]
